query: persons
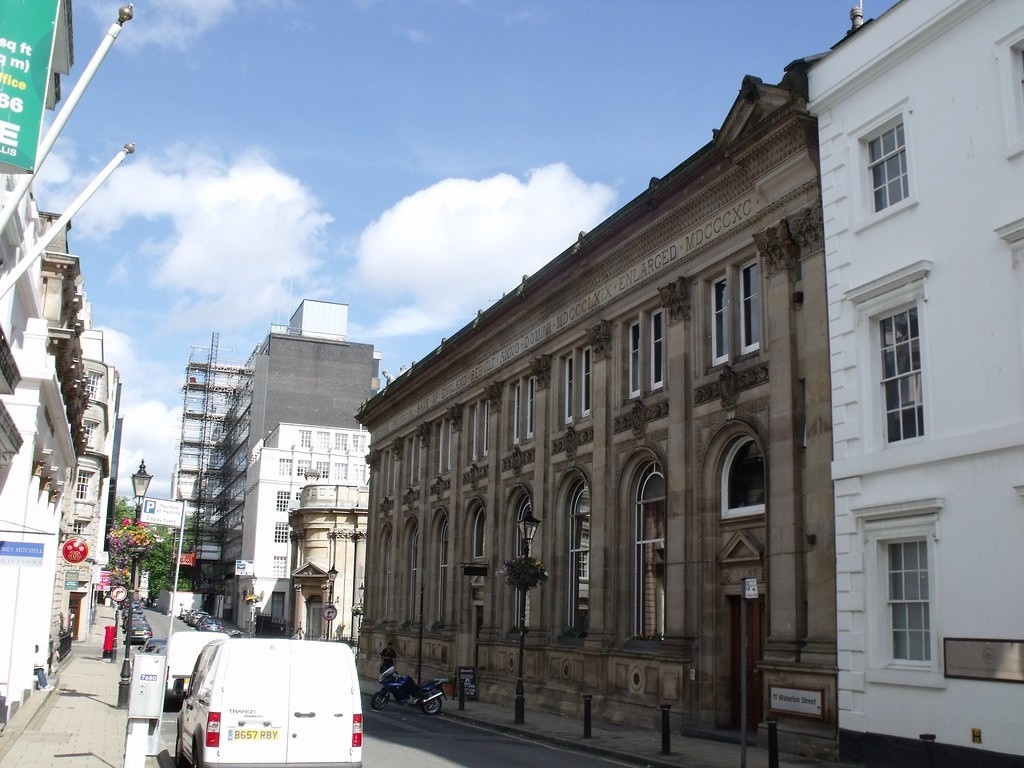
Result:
[380,642,396,675]
[292,628,305,640]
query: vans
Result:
[163,630,232,710]
[171,638,363,768]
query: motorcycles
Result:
[370,665,448,714]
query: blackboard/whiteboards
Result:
[458,666,476,698]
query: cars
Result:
[119,599,153,645]
[180,607,224,633]
[138,638,167,657]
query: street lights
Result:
[326,563,339,641]
[514,509,541,722]
[117,458,155,709]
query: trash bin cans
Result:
[105,596,110,606]
[102,625,117,657]
